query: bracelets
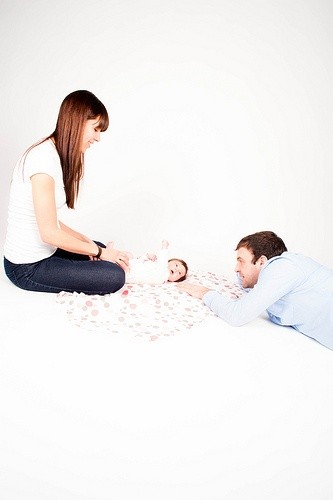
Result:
[93,246,102,257]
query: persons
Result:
[176,231,333,352]
[1,88,130,295]
[106,243,189,286]
